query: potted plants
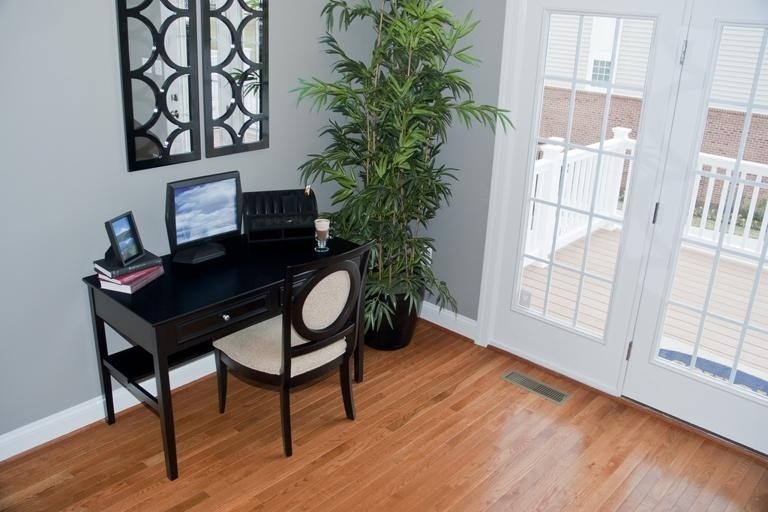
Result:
[314,0,472,349]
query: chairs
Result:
[211,242,372,458]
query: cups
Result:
[313,217,330,253]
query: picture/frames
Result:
[106,211,144,268]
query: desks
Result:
[82,238,380,481]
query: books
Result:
[93,248,165,296]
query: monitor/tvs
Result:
[165,171,243,265]
